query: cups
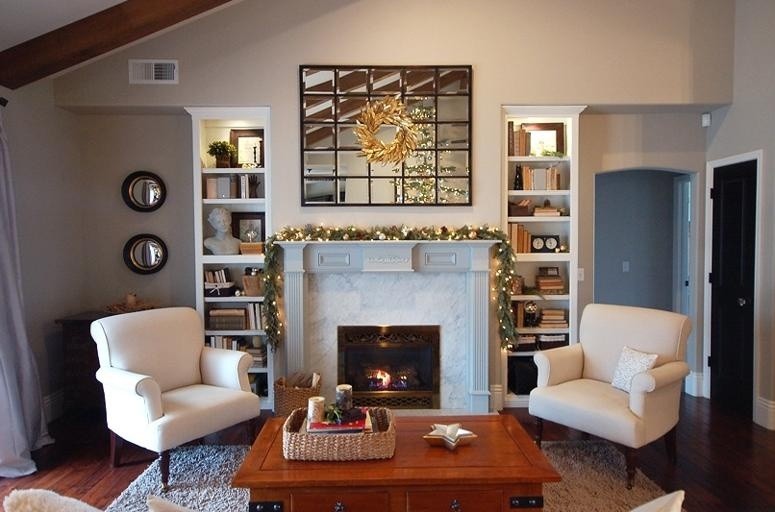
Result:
[127,293,136,306]
[308,396,326,422]
[336,384,353,411]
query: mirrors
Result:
[299,64,473,208]
[122,234,168,275]
[120,171,167,213]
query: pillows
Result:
[2,488,102,512]
[145,494,195,511]
[611,345,659,395]
[630,487,686,512]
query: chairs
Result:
[527,302,693,489]
[90,305,260,492]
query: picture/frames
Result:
[230,209,265,253]
[519,122,565,158]
[229,127,265,169]
[302,164,351,204]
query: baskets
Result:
[282,406,396,461]
[273,378,321,417]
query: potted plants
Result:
[206,140,237,169]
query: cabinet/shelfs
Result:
[182,104,276,413]
[53,309,152,444]
[500,101,587,408]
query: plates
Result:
[104,301,158,313]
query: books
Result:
[201,174,267,368]
[507,120,570,351]
[304,409,373,434]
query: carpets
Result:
[104,415,686,512]
